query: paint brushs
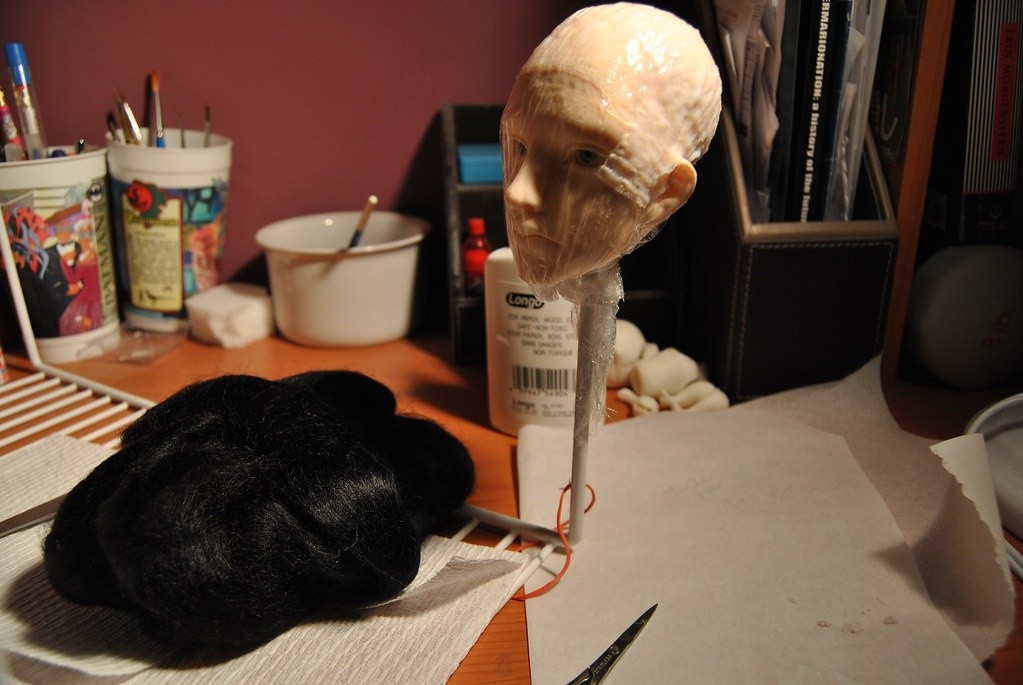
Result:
[103,63,218,147]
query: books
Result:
[783,0,851,225]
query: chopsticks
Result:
[1000,535,1023,579]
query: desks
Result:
[0,328,1023,685]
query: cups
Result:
[105,128,233,333]
[0,145,121,367]
[485,247,606,437]
[253,210,425,348]
[965,393,1023,543]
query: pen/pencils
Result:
[346,193,379,248]
[0,40,88,163]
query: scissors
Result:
[563,599,658,685]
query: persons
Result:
[501,6,724,288]
